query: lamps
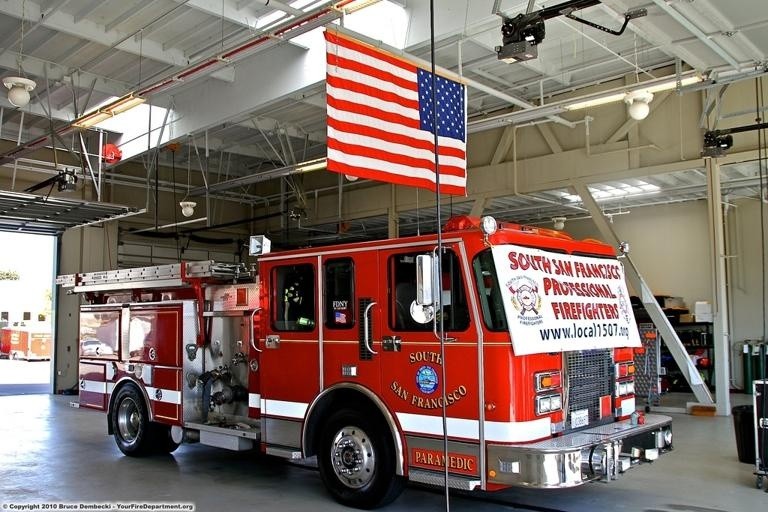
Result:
[621,33,654,123]
[552,216,567,231]
[2,1,37,109]
[178,136,197,218]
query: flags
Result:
[323,32,468,196]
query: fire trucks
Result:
[55,215,674,510]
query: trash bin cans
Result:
[732,404,756,465]
